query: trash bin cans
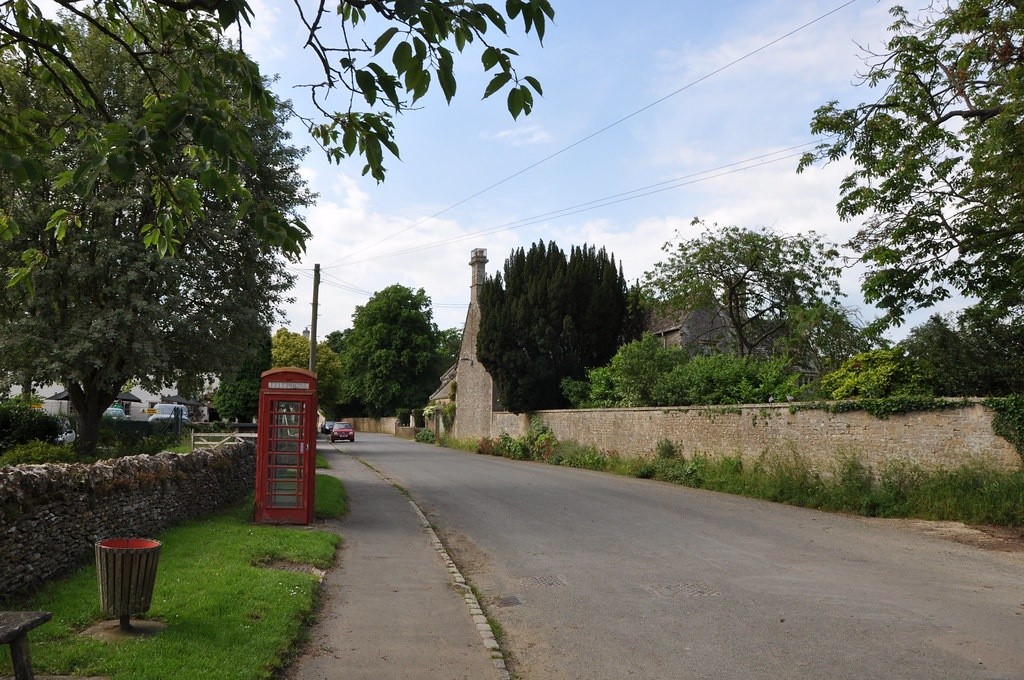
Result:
[94,535,164,616]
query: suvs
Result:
[147,403,189,422]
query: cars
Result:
[321,421,335,434]
[329,422,355,443]
[102,408,131,420]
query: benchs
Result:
[0,610,53,680]
